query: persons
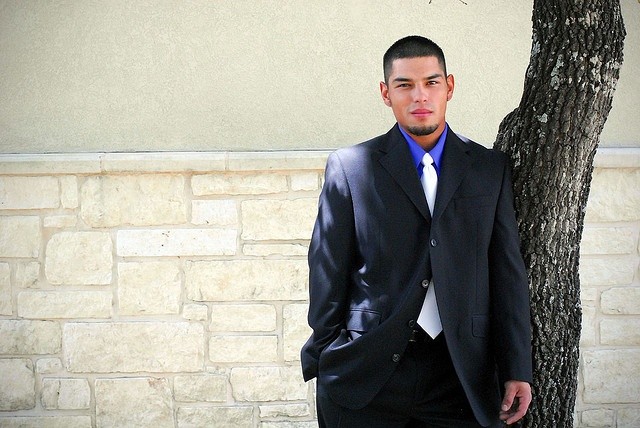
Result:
[300,36,534,428]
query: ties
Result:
[416,153,444,339]
